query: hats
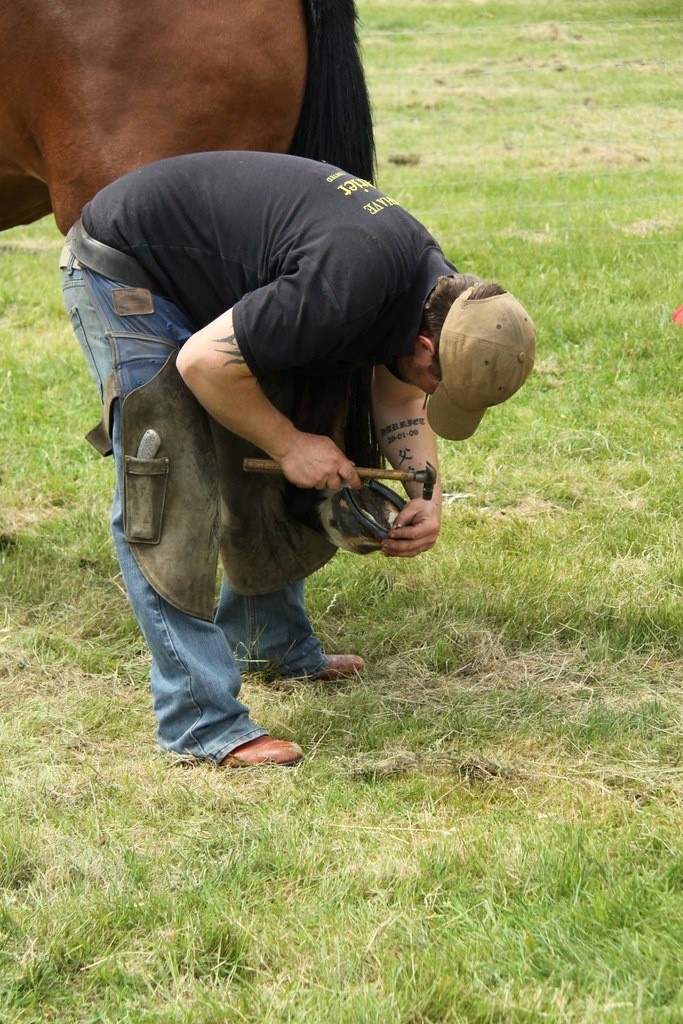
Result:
[425,282,539,441]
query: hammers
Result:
[243,458,437,500]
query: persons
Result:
[60,152,535,767]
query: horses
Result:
[1,0,422,557]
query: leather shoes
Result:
[157,731,303,767]
[242,653,367,683]
